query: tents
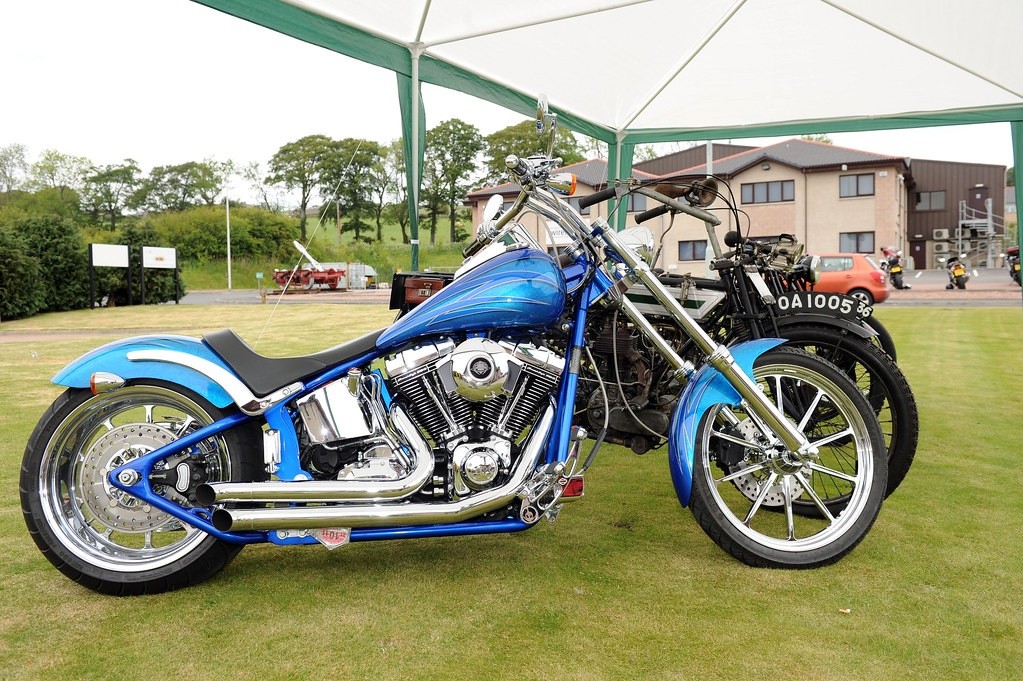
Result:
[192,0,1023,273]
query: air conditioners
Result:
[932,242,949,254]
[954,241,971,252]
[932,253,950,263]
[932,263,948,269]
[933,228,949,240]
[954,229,971,239]
[978,240,996,253]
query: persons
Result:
[881,248,902,267]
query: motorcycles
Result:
[1000,246,1023,287]
[879,243,905,289]
[389,174,919,520]
[939,254,968,290]
[18,93,889,596]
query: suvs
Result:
[768,253,889,308]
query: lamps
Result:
[761,163,770,170]
